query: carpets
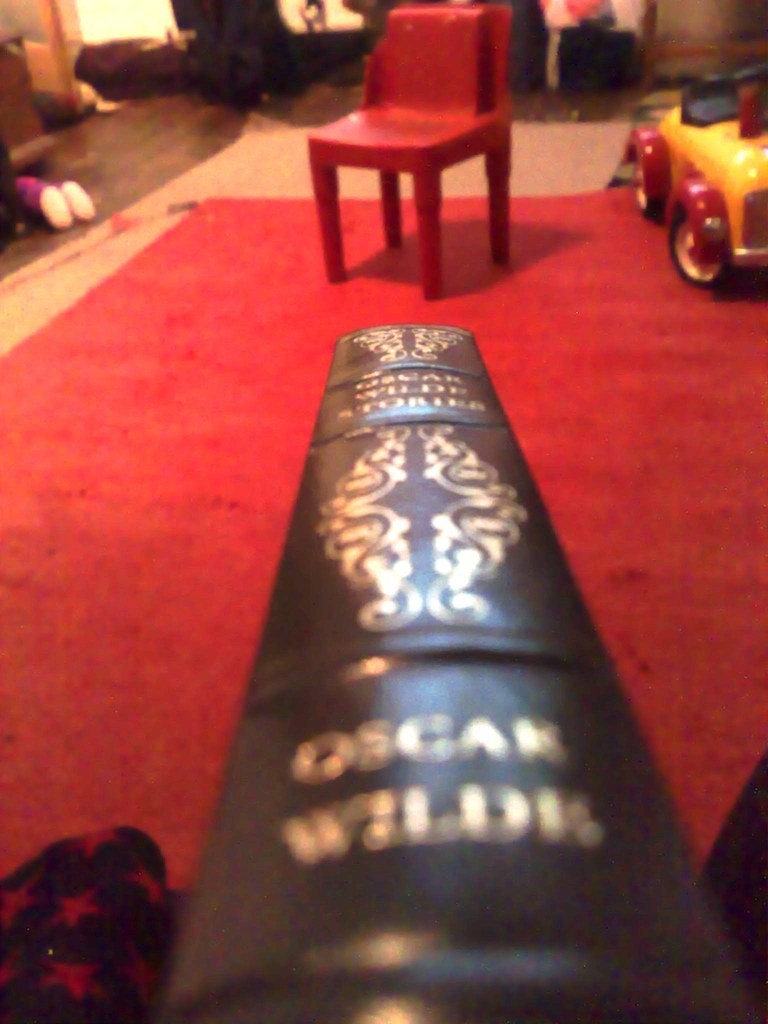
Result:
[0,190,768,1024]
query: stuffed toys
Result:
[10,174,97,230]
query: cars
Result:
[621,61,768,291]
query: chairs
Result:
[306,4,511,300]
